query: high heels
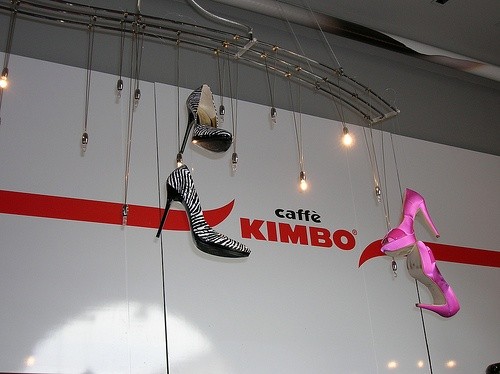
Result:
[157,165,252,258]
[407,241,460,318]
[381,188,440,256]
[180,84,233,153]
